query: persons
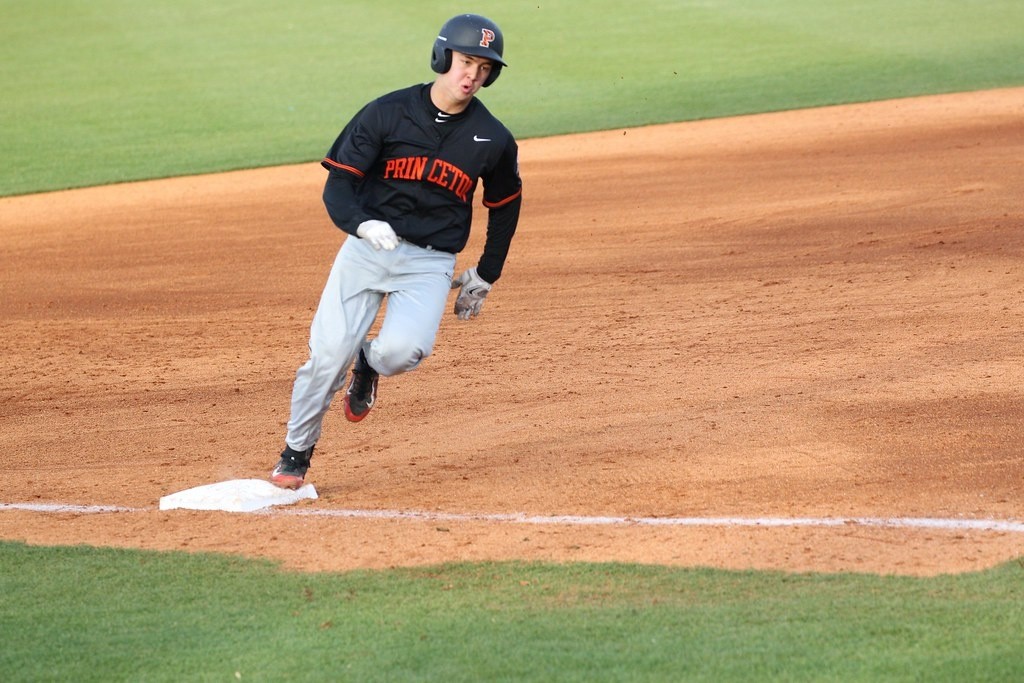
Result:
[271,14,523,493]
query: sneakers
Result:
[270,444,316,490]
[343,347,380,423]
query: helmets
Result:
[431,13,508,88]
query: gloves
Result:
[356,220,402,251]
[450,266,492,322]
